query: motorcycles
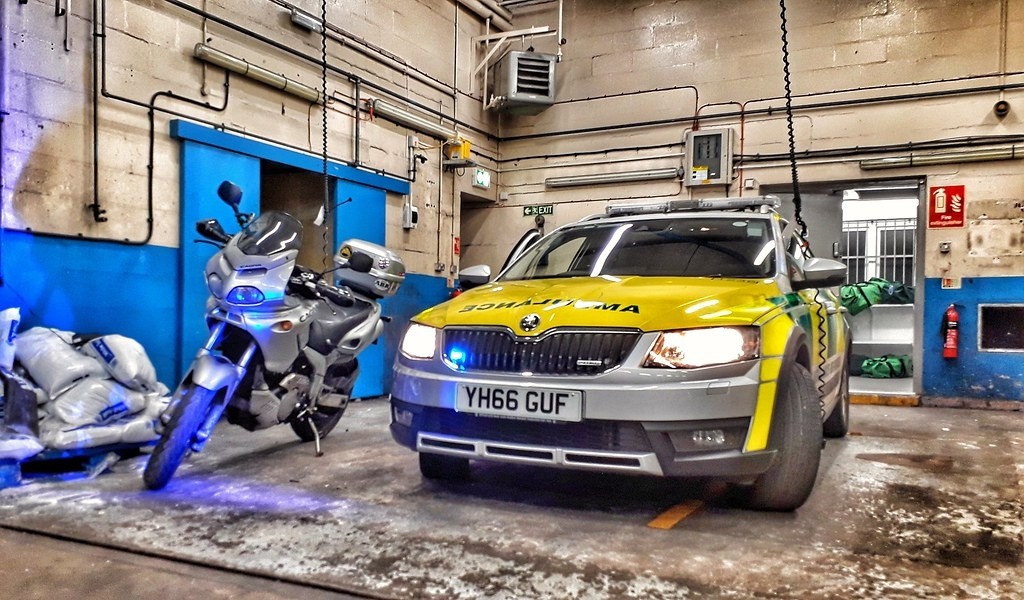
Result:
[144,180,406,491]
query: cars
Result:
[388,194,853,511]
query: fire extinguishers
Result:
[940,304,966,357]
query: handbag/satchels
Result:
[860,353,913,379]
[841,276,894,317]
